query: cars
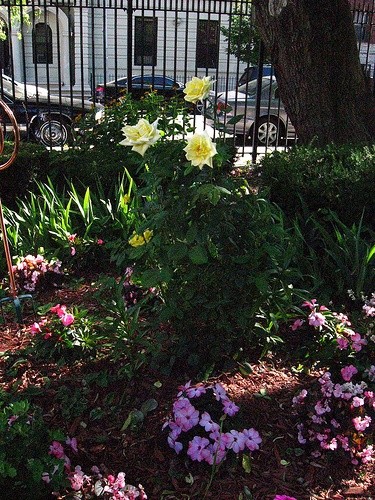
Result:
[200,73,300,147]
[0,74,105,148]
[91,74,214,115]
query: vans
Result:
[239,64,275,88]
